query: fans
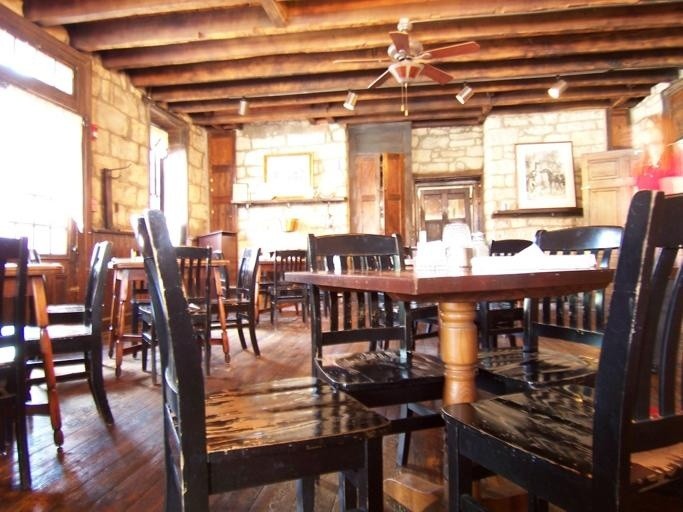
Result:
[330,13,480,97]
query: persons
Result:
[627,112,682,199]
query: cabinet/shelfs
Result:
[574,147,640,305]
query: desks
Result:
[274,261,681,511]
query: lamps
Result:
[384,61,432,118]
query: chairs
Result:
[301,227,449,466]
[466,223,628,414]
[434,189,682,510]
[128,206,392,510]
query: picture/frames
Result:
[512,139,578,212]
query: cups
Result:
[420,222,489,270]
